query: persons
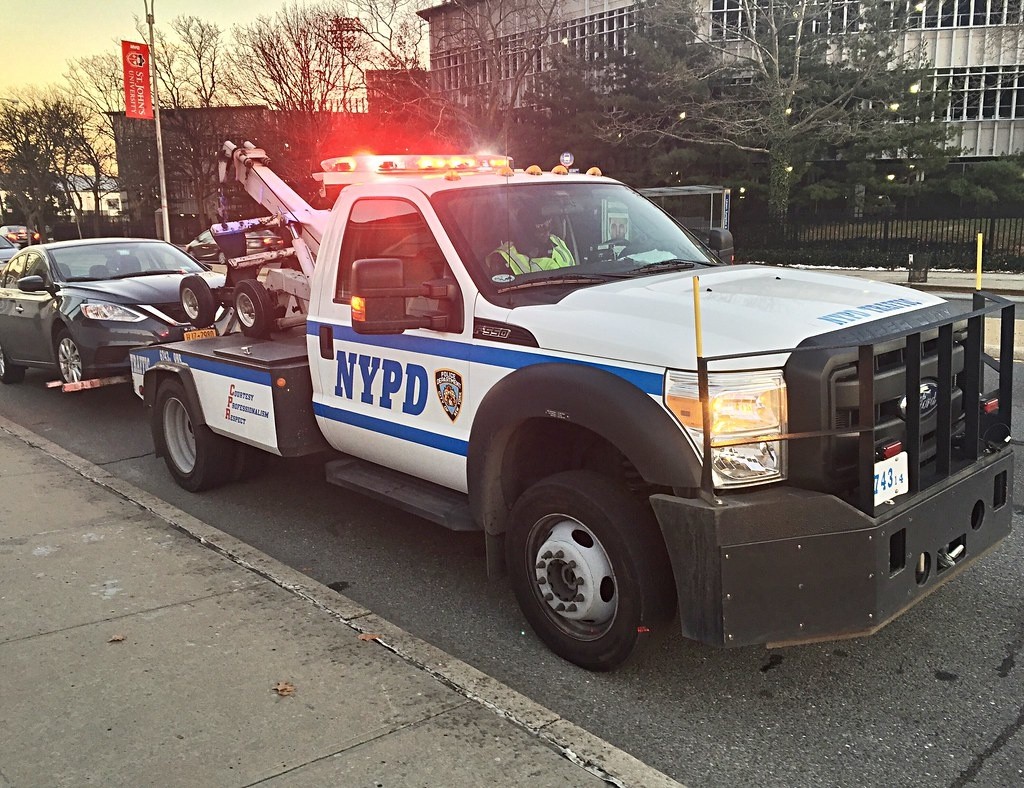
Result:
[484,201,577,280]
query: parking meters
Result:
[709,227,734,268]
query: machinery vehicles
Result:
[128,134,1023,678]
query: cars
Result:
[0,232,23,267]
[184,225,284,267]
[0,236,229,383]
[0,225,40,251]
